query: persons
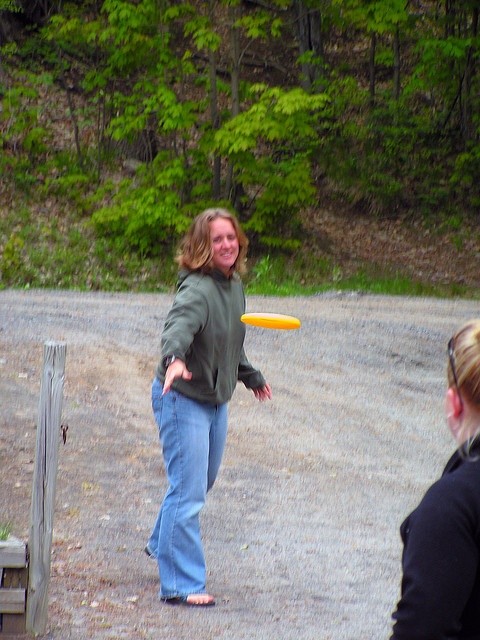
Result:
[388,321,480,640]
[143,208,272,607]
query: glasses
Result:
[447,337,463,418]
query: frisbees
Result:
[240,312,301,329]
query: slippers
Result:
[161,589,216,607]
